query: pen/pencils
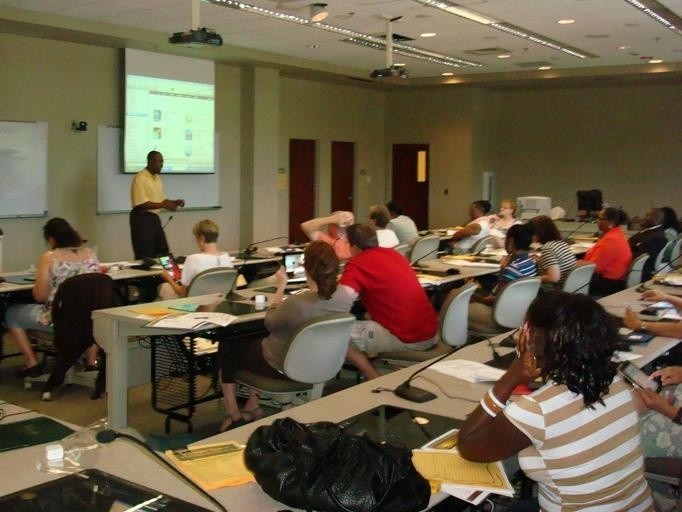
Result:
[23,278,35,281]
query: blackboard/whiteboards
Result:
[96,125,222,215]
[0,120,50,219]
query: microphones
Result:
[93,429,229,511]
[564,215,598,244]
[410,242,447,268]
[498,271,601,349]
[635,254,681,293]
[229,246,257,294]
[162,214,173,230]
[394,334,503,403]
[242,236,300,254]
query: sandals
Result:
[221,406,264,432]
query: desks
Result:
[1,399,226,512]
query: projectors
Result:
[168,27,223,50]
[370,66,407,78]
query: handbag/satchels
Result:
[244,417,431,511]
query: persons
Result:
[129,151,184,261]
[439,197,682,511]
[219,200,439,433]
[159,220,234,302]
[5,216,103,378]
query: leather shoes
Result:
[84,359,101,371]
[13,364,43,377]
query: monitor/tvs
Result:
[575,190,603,218]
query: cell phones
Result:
[615,360,662,395]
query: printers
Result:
[518,195,553,220]
